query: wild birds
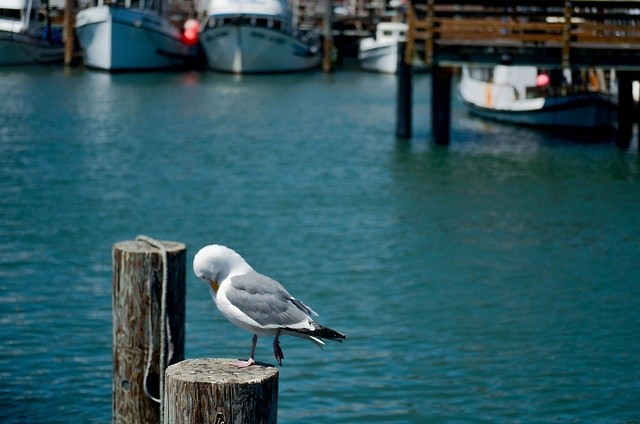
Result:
[193,244,347,369]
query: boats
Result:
[456,61,619,131]
[73,0,199,71]
[200,0,337,73]
[0,0,66,65]
[357,21,427,74]
